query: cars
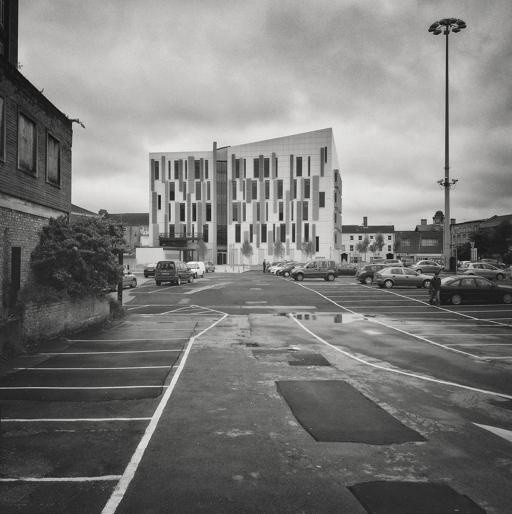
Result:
[110,260,216,290]
[352,255,512,289]
[430,272,512,305]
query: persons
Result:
[428,268,441,305]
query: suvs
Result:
[267,257,339,282]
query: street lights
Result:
[428,15,470,273]
